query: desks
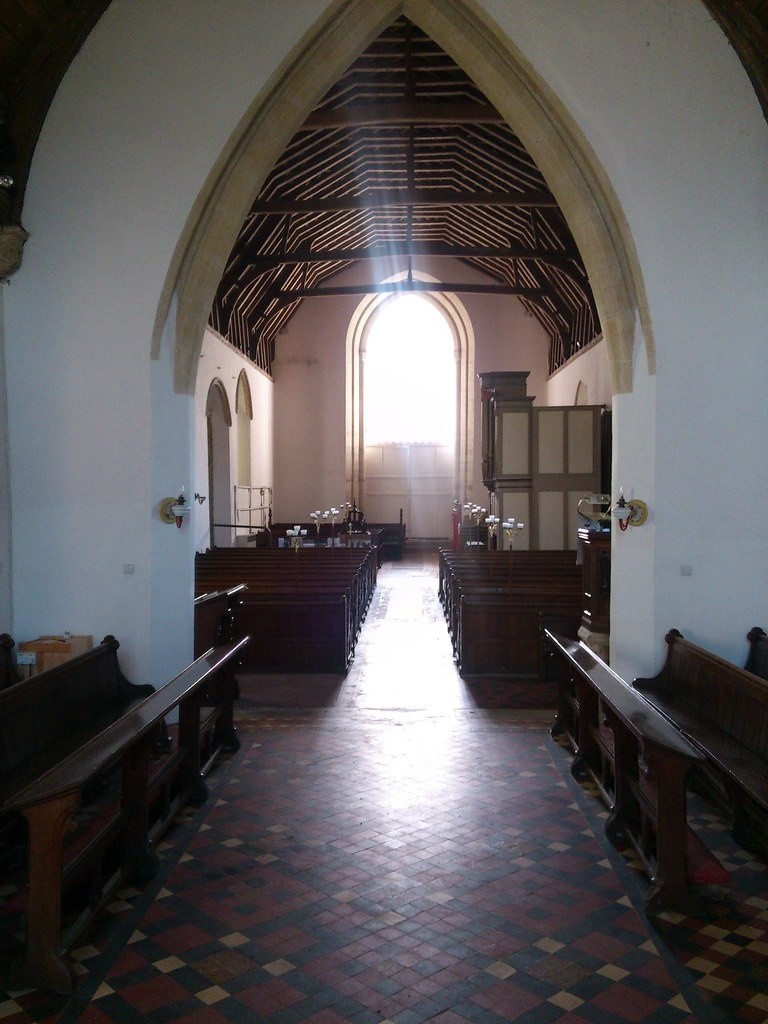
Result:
[0,635,251,996]
[338,528,385,568]
[545,627,721,914]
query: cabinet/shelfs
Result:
[17,635,93,681]
[578,529,610,634]
[476,372,603,550]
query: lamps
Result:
[159,484,191,529]
[309,511,327,526]
[577,494,612,531]
[325,508,339,519]
[340,502,352,512]
[463,501,486,522]
[501,518,524,536]
[612,486,648,531]
[484,514,500,531]
[285,526,307,543]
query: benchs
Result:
[438,547,582,676]
[632,626,768,863]
[193,522,406,674]
[0,632,175,808]
